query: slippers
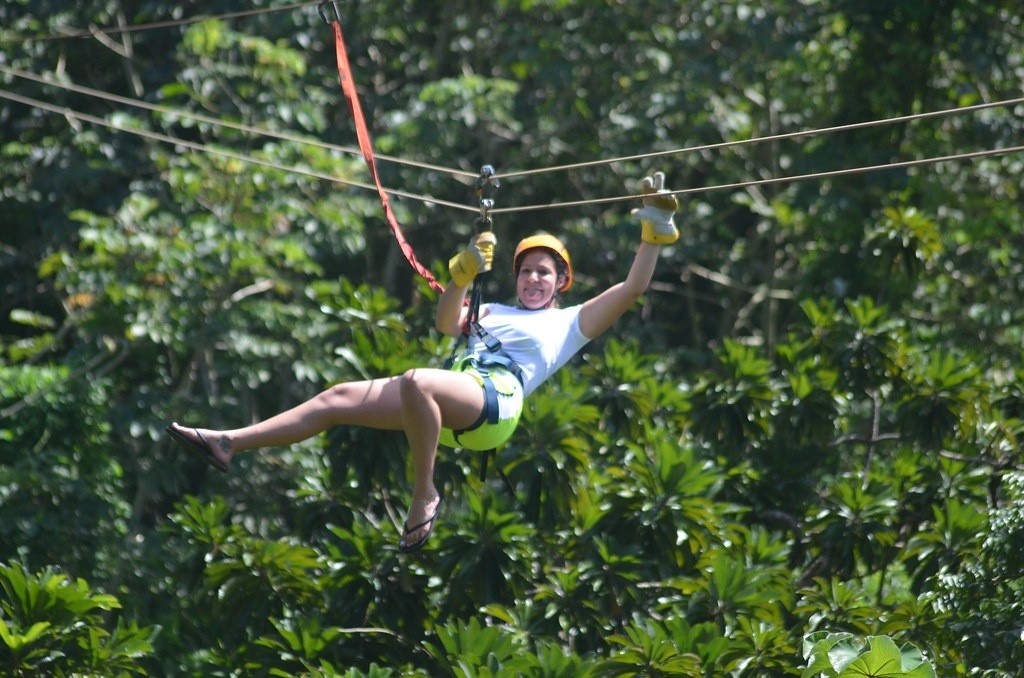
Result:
[165,427,228,473]
[398,493,442,553]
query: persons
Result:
[166,170,679,550]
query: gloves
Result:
[630,171,680,244]
[449,231,498,289]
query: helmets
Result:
[513,232,573,292]
